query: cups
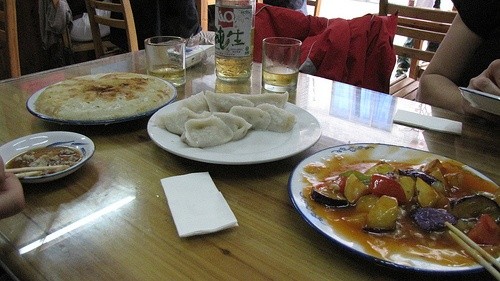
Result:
[144,36,186,87]
[261,37,302,93]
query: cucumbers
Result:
[311,189,348,206]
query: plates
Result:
[26,72,178,127]
[460,86,500,114]
[0,131,96,183]
[288,143,500,272]
[147,91,322,166]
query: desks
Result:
[193,0,263,33]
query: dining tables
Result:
[0,40,500,281]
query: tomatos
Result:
[465,213,500,244]
[370,174,407,205]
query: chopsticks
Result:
[4,165,67,177]
[445,221,500,281]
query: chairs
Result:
[254,3,398,95]
[307,0,322,16]
[85,0,138,58]
[50,0,117,66]
[379,0,459,103]
[0,0,21,80]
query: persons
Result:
[395,0,442,78]
[416,0,500,115]
[109,0,201,49]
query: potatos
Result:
[342,157,466,232]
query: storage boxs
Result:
[167,42,215,67]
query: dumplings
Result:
[160,89,295,148]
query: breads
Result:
[34,72,175,121]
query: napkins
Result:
[393,110,463,137]
[159,171,238,238]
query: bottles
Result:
[213,0,256,82]
